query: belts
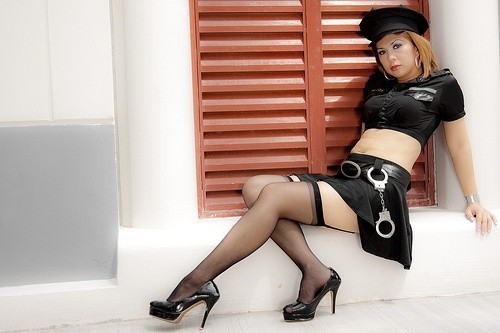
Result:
[341,159,411,190]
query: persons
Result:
[150,7,498,329]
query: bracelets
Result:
[466,194,481,204]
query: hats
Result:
[359,7,429,41]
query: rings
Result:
[481,220,487,224]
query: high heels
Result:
[283,268,341,321]
[149,279,220,328]
